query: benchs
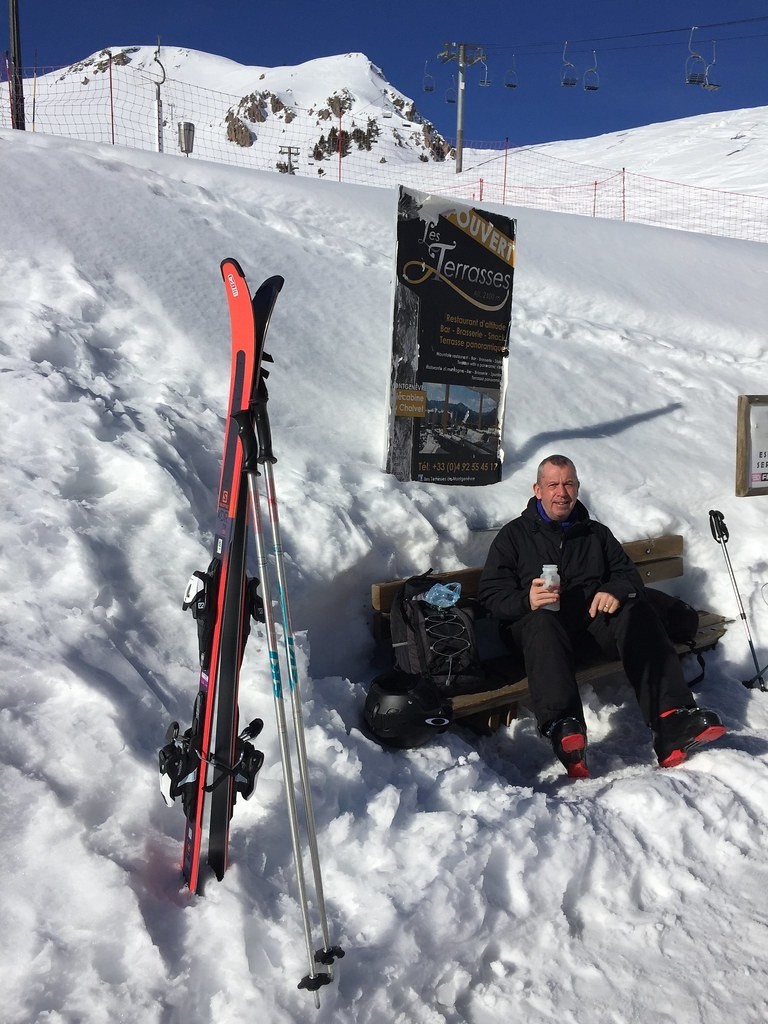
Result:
[372,535,738,736]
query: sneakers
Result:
[552,717,589,779]
[653,705,726,767]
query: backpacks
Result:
[640,587,698,642]
[390,569,478,679]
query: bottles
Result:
[539,565,561,611]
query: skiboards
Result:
[181,257,289,897]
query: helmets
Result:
[364,668,450,750]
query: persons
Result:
[479,455,726,778]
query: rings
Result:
[605,605,610,609]
